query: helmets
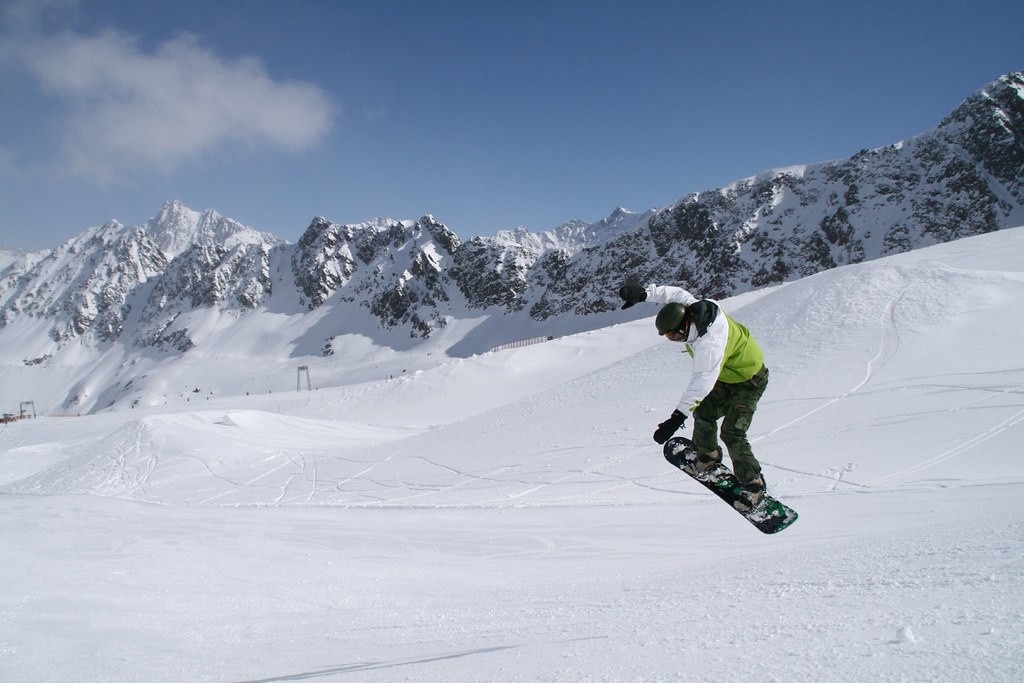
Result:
[655,302,686,334]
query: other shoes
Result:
[734,476,765,510]
[684,447,722,474]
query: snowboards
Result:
[663,436,800,535]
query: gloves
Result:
[619,286,647,310]
[653,410,687,444]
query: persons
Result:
[618,283,768,510]
